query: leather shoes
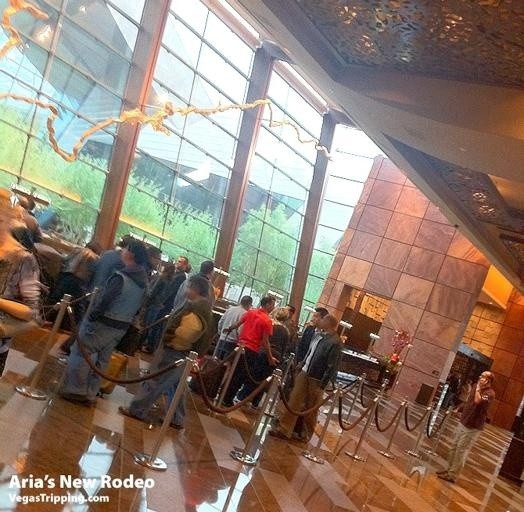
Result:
[436,470,456,483]
[160,416,183,430]
[62,393,95,408]
[269,430,309,442]
[119,406,145,420]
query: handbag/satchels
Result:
[166,311,208,352]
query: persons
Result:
[434,371,497,484]
[452,381,471,413]
[0,188,342,444]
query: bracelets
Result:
[476,388,480,392]
